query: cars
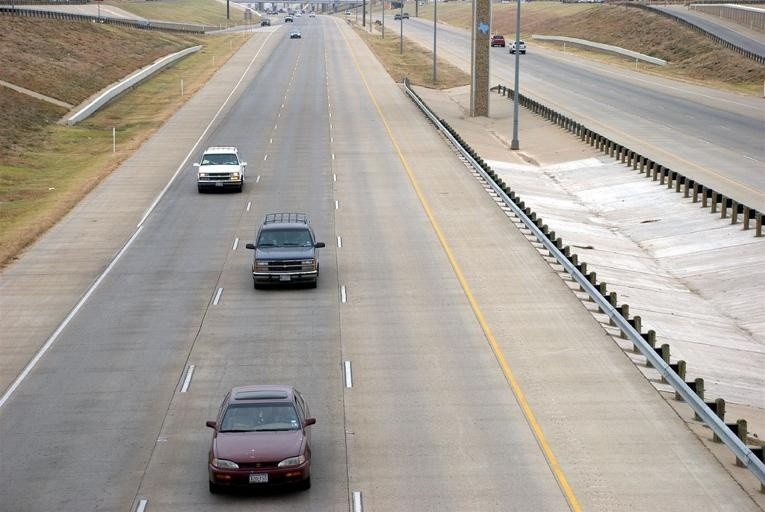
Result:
[402,12,410,18]
[289,28,302,39]
[394,13,401,20]
[491,33,506,48]
[345,9,350,15]
[258,4,319,26]
[204,381,320,497]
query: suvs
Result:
[190,143,248,195]
[507,40,527,55]
[243,210,327,294]
